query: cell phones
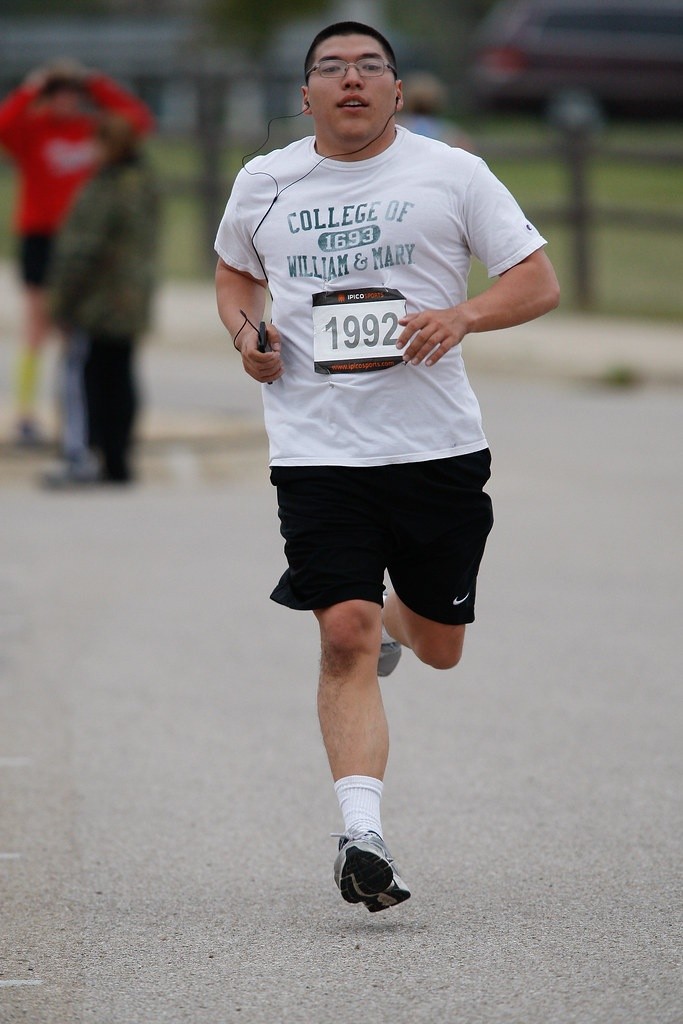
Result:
[257,322,273,385]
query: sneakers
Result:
[333,829,410,911]
[378,593,401,677]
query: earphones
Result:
[396,96,400,106]
[306,101,309,107]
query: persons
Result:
[209,21,565,918]
[38,114,165,485]
[4,60,153,448]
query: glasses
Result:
[304,59,399,86]
